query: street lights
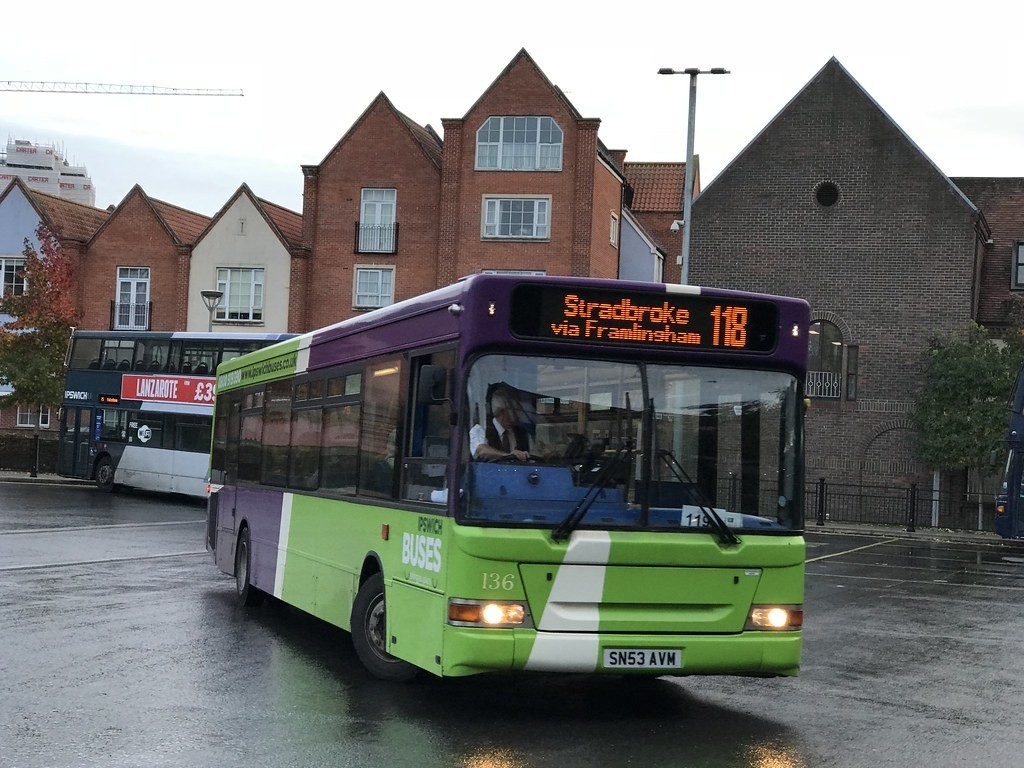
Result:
[656,66,730,287]
[200,289,224,332]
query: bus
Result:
[53,328,309,503]
[991,365,1024,541]
[202,272,816,687]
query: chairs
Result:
[214,420,394,499]
[86,355,217,377]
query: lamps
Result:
[985,239,994,246]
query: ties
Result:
[502,429,511,453]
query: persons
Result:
[386,430,395,470]
[469,390,555,465]
